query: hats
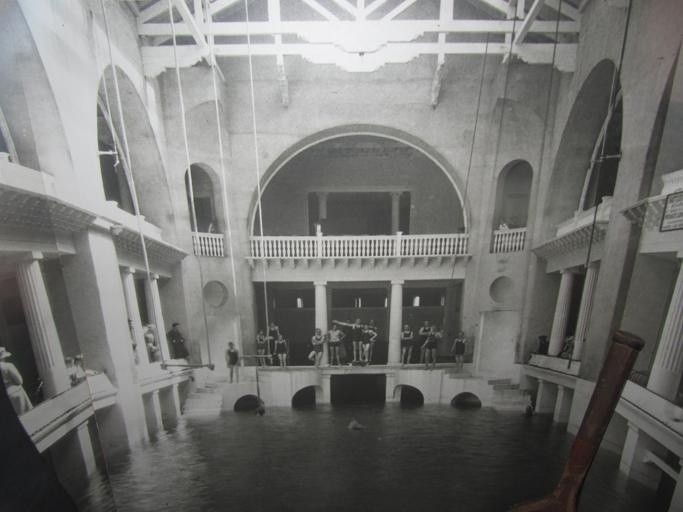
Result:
[0,347,12,359]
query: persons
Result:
[143,322,188,359]
[225,343,239,384]
[65,354,85,386]
[450,331,467,371]
[256,322,288,368]
[401,320,441,370]
[0,345,32,417]
[308,318,378,366]
